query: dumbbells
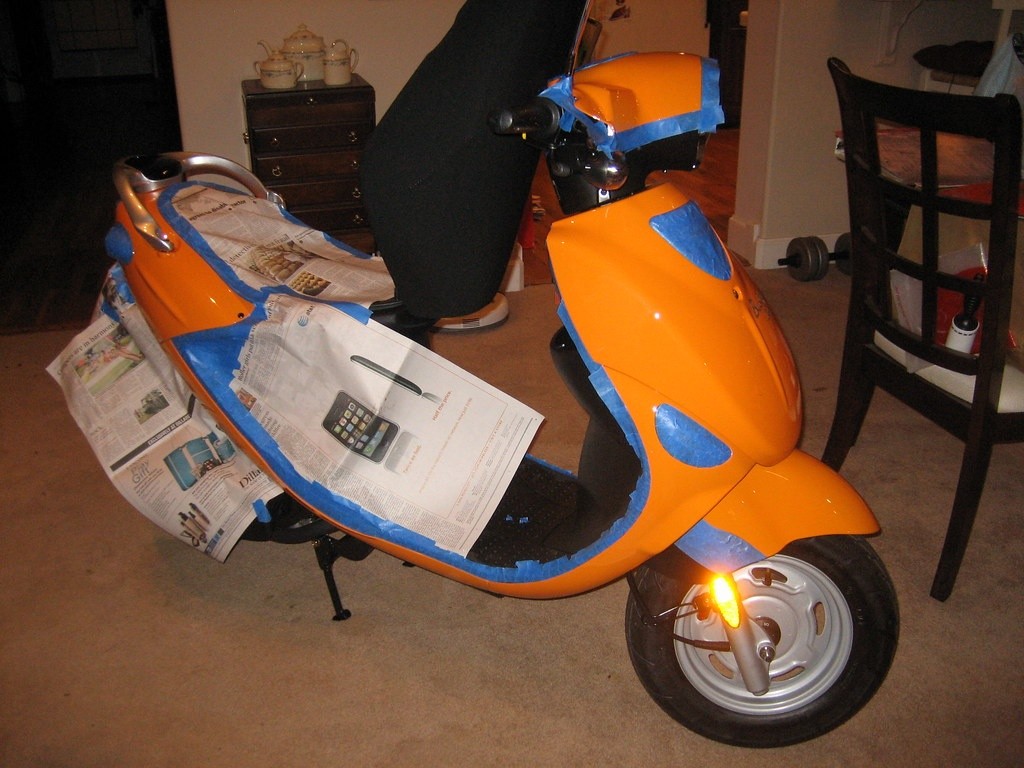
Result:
[777,232,854,282]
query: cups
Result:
[324,48,360,86]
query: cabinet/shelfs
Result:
[241,71,376,238]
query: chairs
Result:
[815,55,1023,603]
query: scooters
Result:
[104,48,900,750]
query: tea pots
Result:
[257,22,348,81]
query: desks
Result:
[835,117,1023,254]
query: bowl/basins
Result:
[253,47,304,90]
